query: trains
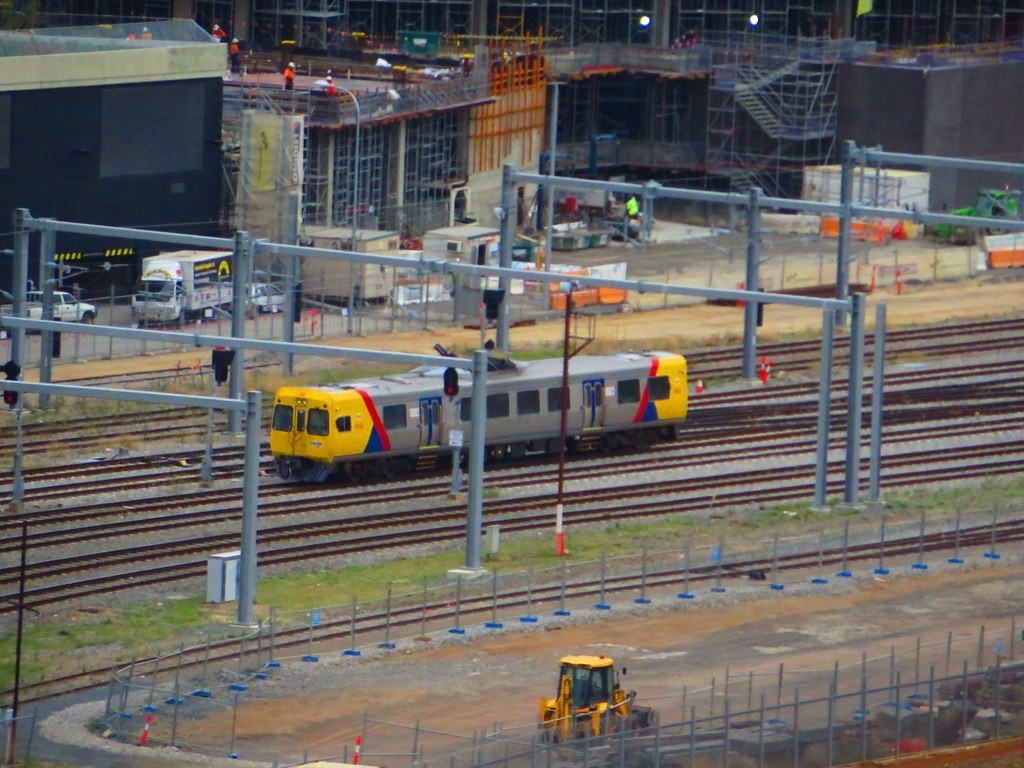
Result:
[267,347,688,486]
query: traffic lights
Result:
[211,350,236,385]
[444,368,460,397]
[4,361,19,404]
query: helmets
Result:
[326,75,332,80]
[213,24,219,30]
[143,27,148,32]
[232,38,239,43]
[288,61,295,67]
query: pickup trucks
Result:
[0,290,97,335]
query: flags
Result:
[856,0,873,18]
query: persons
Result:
[230,37,243,76]
[670,29,697,51]
[626,193,639,220]
[141,26,153,40]
[284,62,296,90]
[324,77,335,96]
[463,58,473,77]
[212,24,226,43]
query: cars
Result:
[245,283,286,313]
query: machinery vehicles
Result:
[538,652,657,749]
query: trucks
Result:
[130,249,233,323]
